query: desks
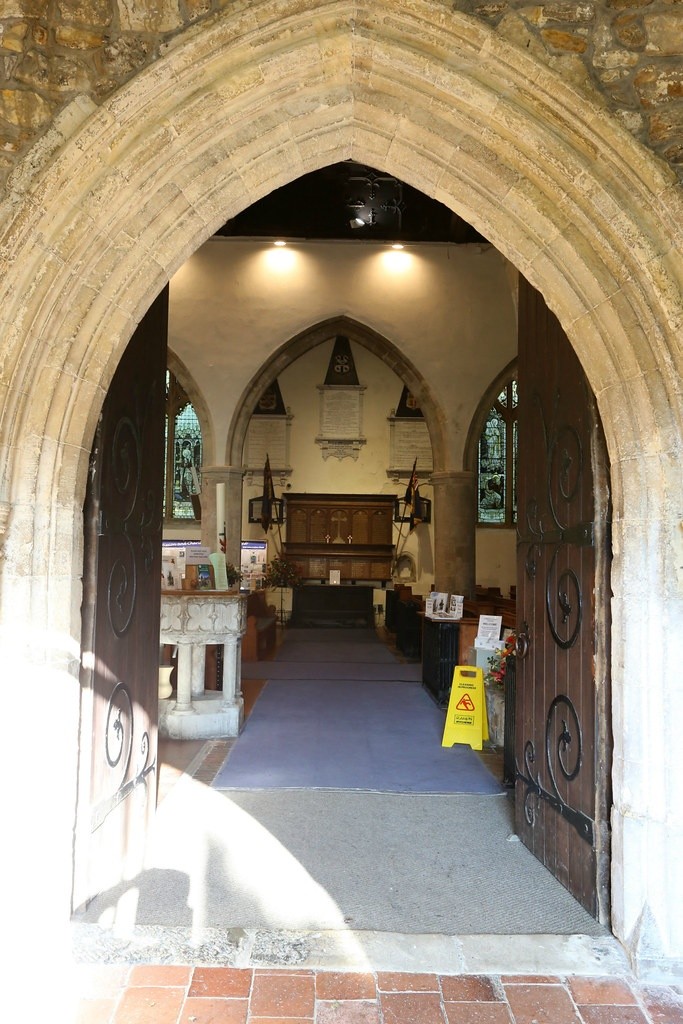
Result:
[284,584,377,628]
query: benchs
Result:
[241,591,278,664]
[386,584,517,652]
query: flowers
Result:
[481,635,514,692]
[258,557,302,590]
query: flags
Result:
[261,461,274,534]
[404,464,422,535]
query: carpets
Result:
[72,630,613,937]
